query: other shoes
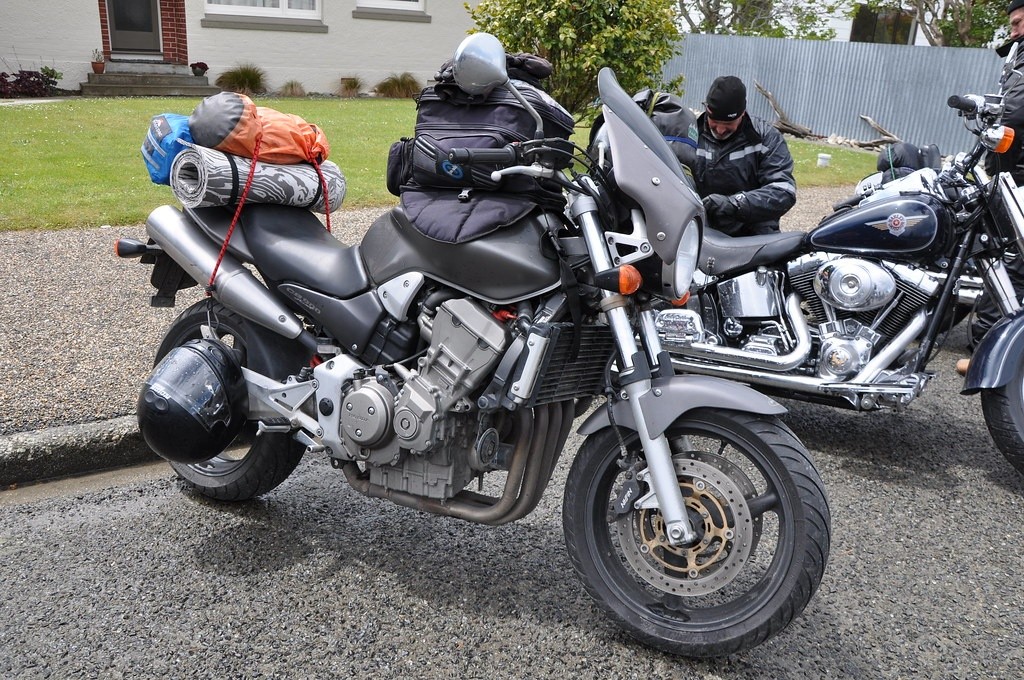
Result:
[955,358,970,376]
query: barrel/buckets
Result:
[817,153,831,167]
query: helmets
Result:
[137,337,250,466]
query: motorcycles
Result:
[115,33,1023,662]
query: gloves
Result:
[701,193,737,221]
[434,51,553,105]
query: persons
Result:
[985,0,1024,188]
[692,75,795,296]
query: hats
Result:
[1006,0,1024,15]
[704,75,747,123]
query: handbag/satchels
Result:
[386,80,575,246]
